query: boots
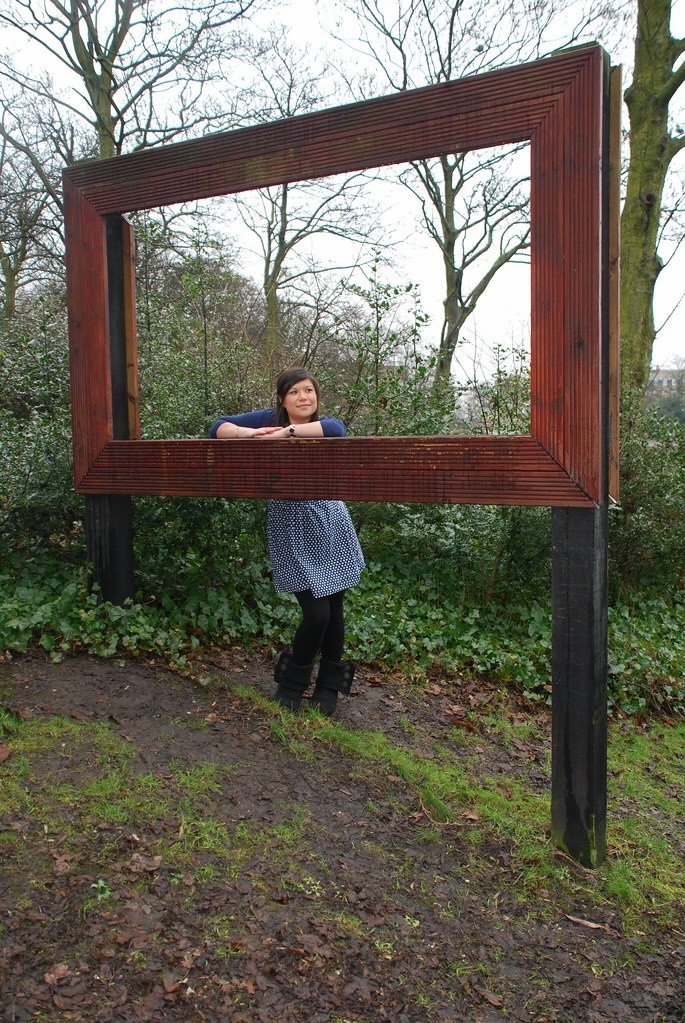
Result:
[313,657,356,716]
[274,652,314,712]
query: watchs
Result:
[289,425,296,437]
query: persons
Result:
[210,368,367,716]
